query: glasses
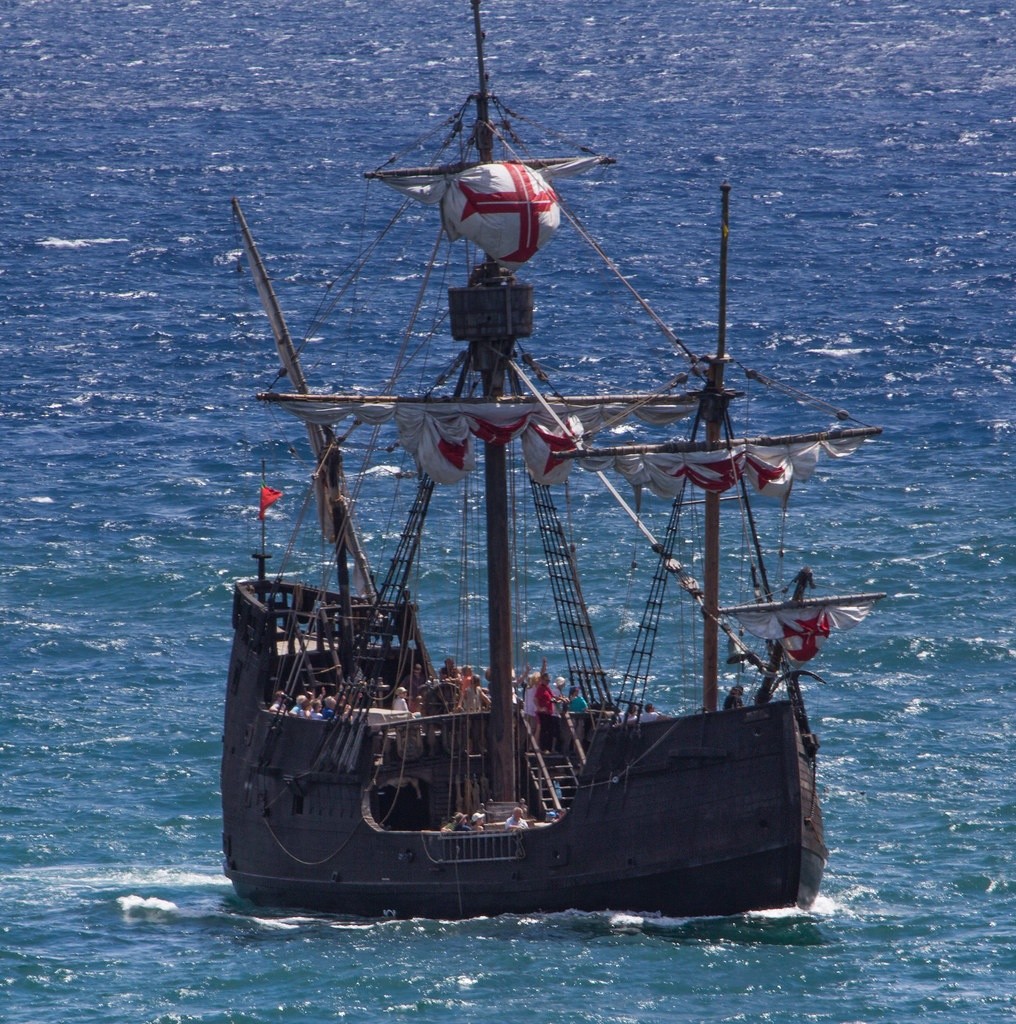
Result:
[545,679,552,682]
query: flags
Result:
[258,483,282,520]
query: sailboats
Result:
[221,3,885,913]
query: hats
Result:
[528,672,540,679]
[553,677,567,686]
[455,813,468,824]
[395,687,408,695]
[732,685,743,695]
[472,813,484,822]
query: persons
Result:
[524,675,540,752]
[463,674,491,712]
[340,704,353,722]
[297,701,311,719]
[534,673,566,753]
[401,664,426,707]
[322,686,343,719]
[289,695,308,717]
[551,676,570,754]
[519,674,528,708]
[568,685,588,712]
[722,685,744,710]
[639,704,659,724]
[439,657,461,682]
[512,663,531,705]
[627,705,639,722]
[460,667,489,694]
[309,699,323,719]
[392,687,422,733]
[269,690,289,717]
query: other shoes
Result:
[544,749,558,755]
[525,752,536,756]
